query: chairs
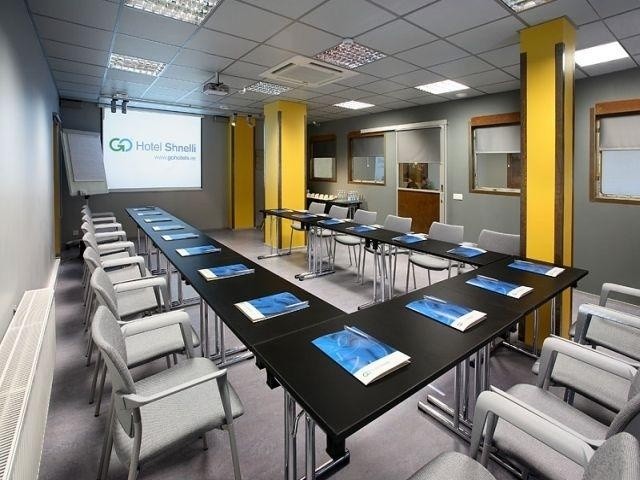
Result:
[307,205,348,263]
[359,215,412,301]
[532,304,640,411]
[569,282,640,362]
[91,305,244,480]
[405,390,640,480]
[84,246,162,366]
[81,222,125,306]
[82,232,141,331]
[81,205,113,217]
[478,336,640,480]
[82,215,122,287]
[333,209,377,281]
[90,268,200,416]
[80,208,116,224]
[478,228,520,256]
[405,222,463,294]
[290,202,326,253]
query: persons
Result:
[407,174,419,189]
[421,176,434,189]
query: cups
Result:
[307,193,334,200]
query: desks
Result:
[125,206,348,370]
[307,196,362,218]
[258,208,509,312]
[253,258,589,480]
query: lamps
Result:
[121,100,128,114]
[110,99,117,113]
[231,112,239,126]
[247,114,252,124]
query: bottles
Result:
[337,190,363,202]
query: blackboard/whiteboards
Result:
[60,128,109,197]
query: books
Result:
[446,245,487,257]
[405,295,487,332]
[392,234,427,244]
[233,292,310,324]
[310,326,412,386]
[507,261,565,278]
[197,264,255,281]
[134,205,222,256]
[271,209,377,233]
[465,275,534,300]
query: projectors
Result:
[203,83,229,96]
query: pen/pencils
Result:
[234,268,255,275]
[205,248,221,252]
[513,259,534,265]
[285,300,309,308]
[445,248,455,253]
[477,274,499,282]
[344,325,369,340]
[423,294,447,304]
